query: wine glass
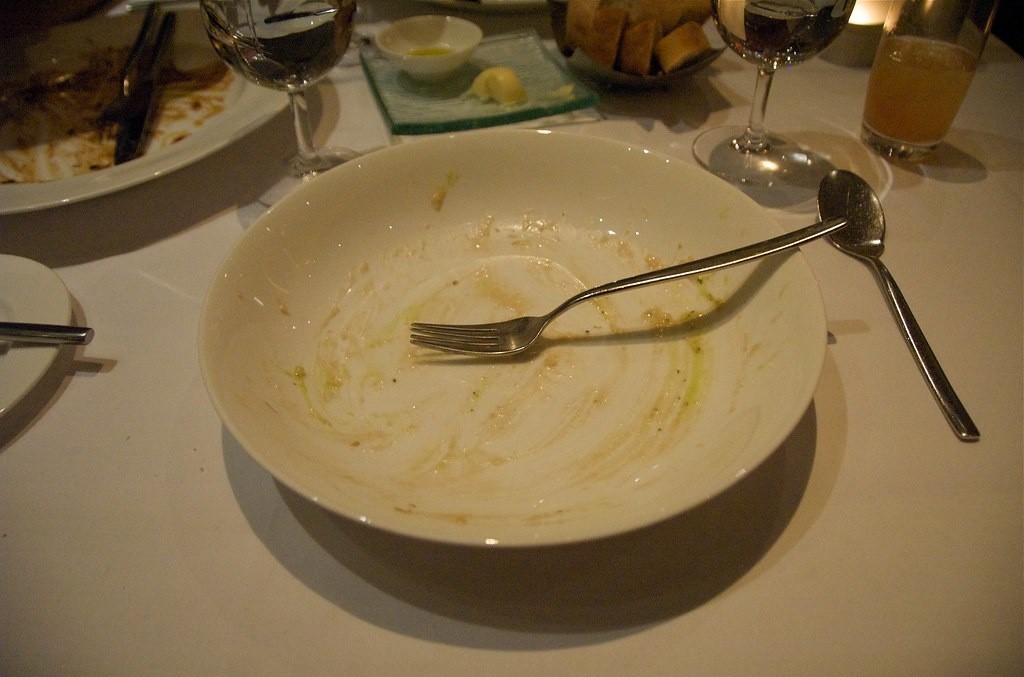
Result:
[198,0,364,209]
[690,0,856,186]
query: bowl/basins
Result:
[374,15,483,82]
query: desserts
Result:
[470,66,526,106]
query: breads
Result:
[565,0,710,75]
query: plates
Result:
[198,124,829,550]
[0,0,290,217]
[0,253,73,419]
[360,26,599,135]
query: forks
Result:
[409,214,851,357]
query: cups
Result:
[859,0,998,162]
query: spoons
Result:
[815,168,982,443]
